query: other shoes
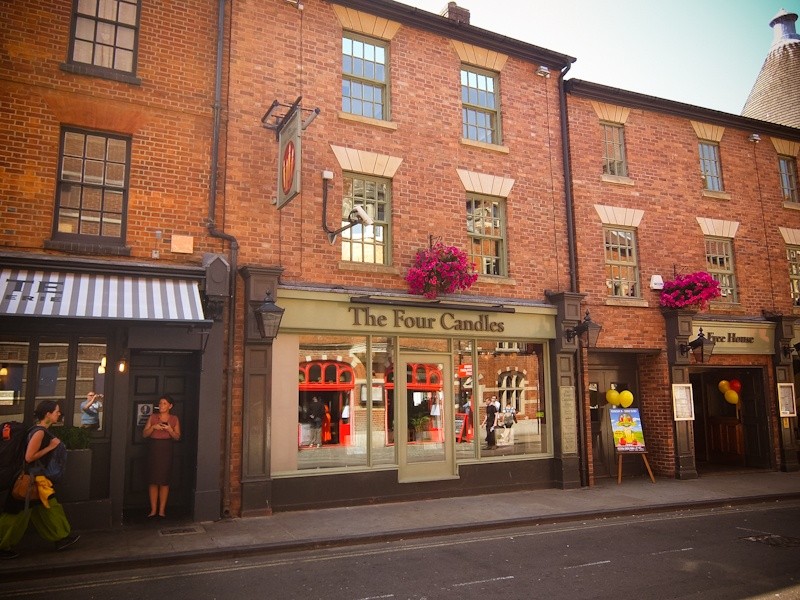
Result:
[0,551,21,560]
[56,534,81,551]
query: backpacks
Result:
[26,426,69,482]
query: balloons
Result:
[725,390,739,405]
[606,389,620,406]
[729,379,740,393]
[719,380,730,393]
[619,391,634,409]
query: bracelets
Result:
[170,431,174,434]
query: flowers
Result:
[401,240,480,299]
[657,271,722,310]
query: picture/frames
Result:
[671,383,695,421]
[777,382,796,417]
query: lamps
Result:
[254,288,286,337]
[566,308,601,348]
[681,326,715,363]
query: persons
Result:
[0,399,80,559]
[500,401,519,445]
[309,395,325,448]
[490,396,501,412]
[482,398,498,450]
[80,392,103,428]
[143,397,180,519]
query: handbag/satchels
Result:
[13,475,40,504]
[0,421,36,491]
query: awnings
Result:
[0,264,214,325]
